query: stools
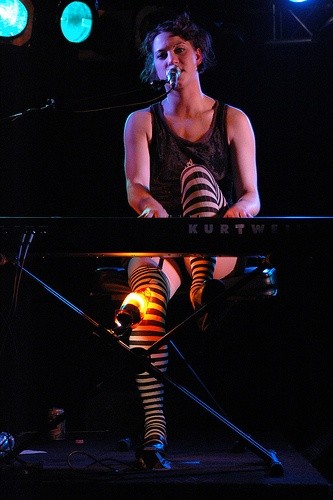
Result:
[95,266,279,460]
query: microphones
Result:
[166,65,180,89]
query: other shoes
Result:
[194,280,227,331]
[137,435,169,463]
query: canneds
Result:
[48,408,65,440]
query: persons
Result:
[123,16,261,463]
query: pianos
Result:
[0,217,333,478]
